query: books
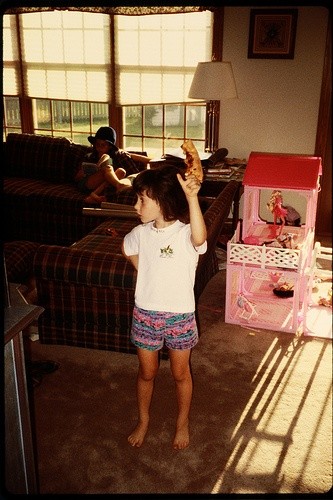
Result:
[205,163,241,178]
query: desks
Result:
[2,301,45,494]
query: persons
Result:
[121,169,207,450]
[73,127,133,203]
[270,190,288,235]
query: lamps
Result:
[190,54,239,153]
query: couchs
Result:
[2,133,237,359]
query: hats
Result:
[88,126,118,152]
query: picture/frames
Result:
[248,8,299,60]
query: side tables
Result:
[203,164,247,232]
